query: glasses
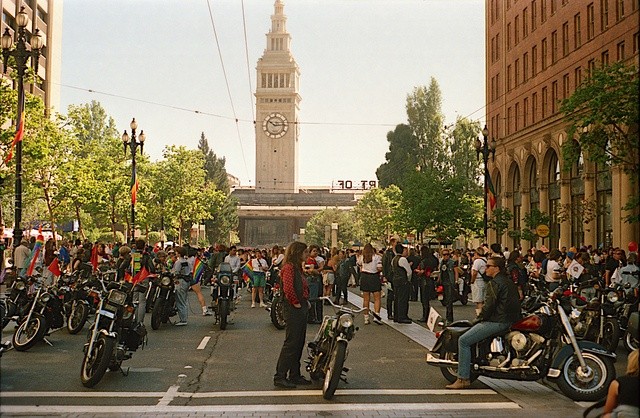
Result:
[615,253,623,255]
[442,253,449,256]
[486,265,496,268]
[219,250,225,253]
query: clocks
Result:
[262,112,289,139]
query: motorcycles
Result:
[79,262,149,385]
[568,281,631,352]
[426,275,616,400]
[266,282,286,329]
[1,258,99,350]
[152,258,191,328]
[304,296,381,398]
[208,262,239,329]
[435,264,470,306]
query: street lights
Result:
[123,119,146,244]
[476,125,497,249]
[3,8,42,249]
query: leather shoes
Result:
[330,301,339,305]
[398,319,412,324]
[341,301,348,305]
[446,379,470,389]
[289,375,312,386]
[420,318,427,322]
[274,378,296,388]
[417,317,421,320]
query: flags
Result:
[485,167,496,209]
[191,257,206,282]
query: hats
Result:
[118,246,132,254]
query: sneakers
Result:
[203,310,213,316]
[176,322,188,326]
[364,318,370,324]
[373,316,383,325]
[251,303,256,308]
[260,304,267,308]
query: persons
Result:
[385,238,397,320]
[170,246,194,326]
[330,250,353,306]
[490,242,504,259]
[469,246,490,317]
[435,246,490,262]
[414,245,434,324]
[505,243,640,259]
[2,236,121,268]
[224,245,240,302]
[194,246,215,289]
[274,240,313,389]
[127,239,156,324]
[389,243,413,324]
[348,246,362,288]
[356,242,385,326]
[183,245,215,315]
[303,244,318,323]
[321,245,341,305]
[315,246,324,324]
[248,248,270,309]
[445,256,522,389]
[435,248,458,323]
[12,240,33,281]
[153,239,174,272]
[114,246,132,282]
[506,250,529,302]
[236,242,254,287]
[544,249,569,292]
[605,247,627,289]
[601,349,639,417]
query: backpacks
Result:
[476,258,493,281]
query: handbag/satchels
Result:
[127,322,148,350]
